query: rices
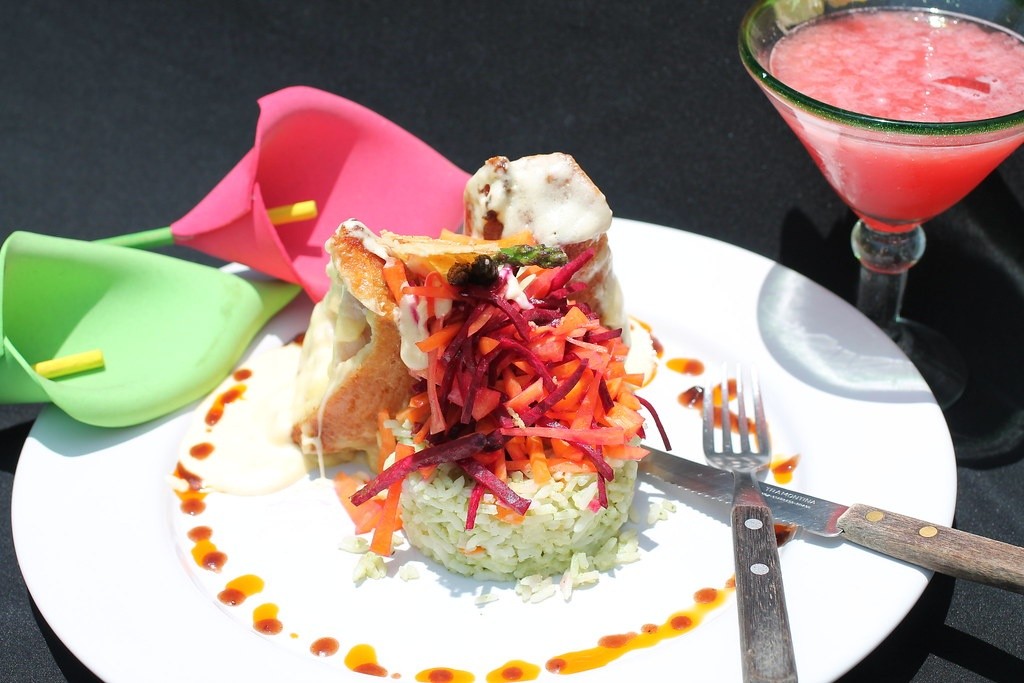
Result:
[378,419,642,606]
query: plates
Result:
[10,214,957,683]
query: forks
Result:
[702,359,802,683]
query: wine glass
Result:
[738,0,1023,411]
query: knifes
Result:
[639,443,1024,596]
